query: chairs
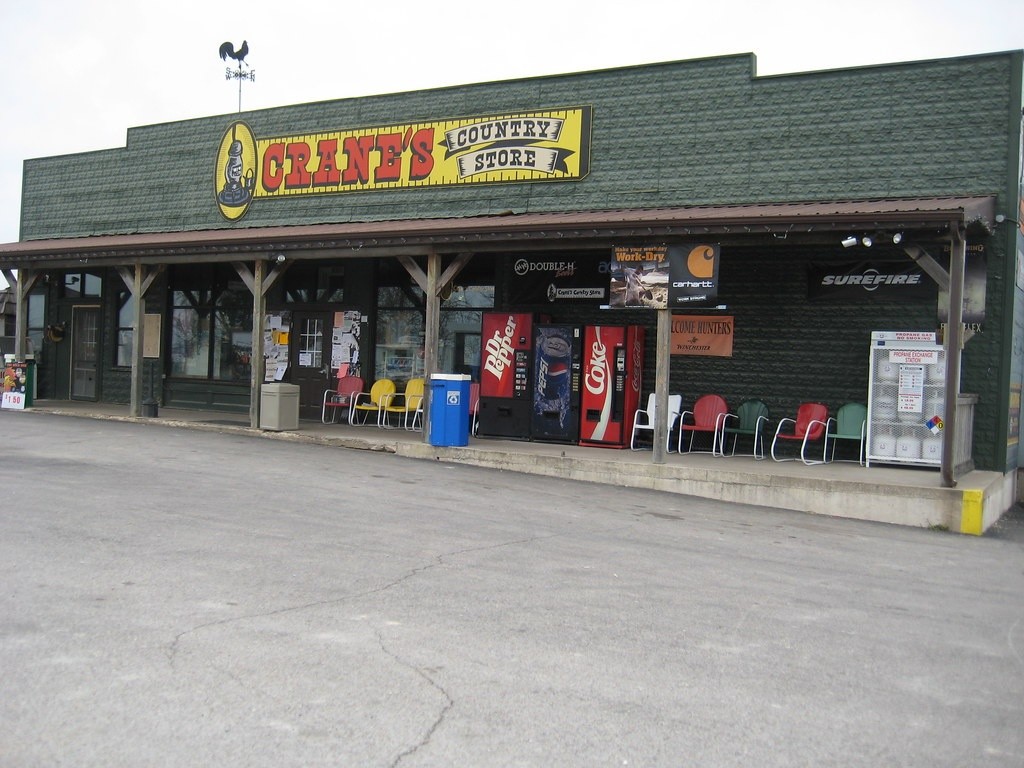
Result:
[321,376,364,425]
[350,379,395,429]
[719,398,770,460]
[678,393,728,458]
[469,383,480,437]
[630,393,682,454]
[770,402,828,466]
[383,378,424,431]
[823,402,867,467]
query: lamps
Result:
[841,230,904,248]
[268,244,286,269]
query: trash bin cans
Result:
[429,373,471,448]
